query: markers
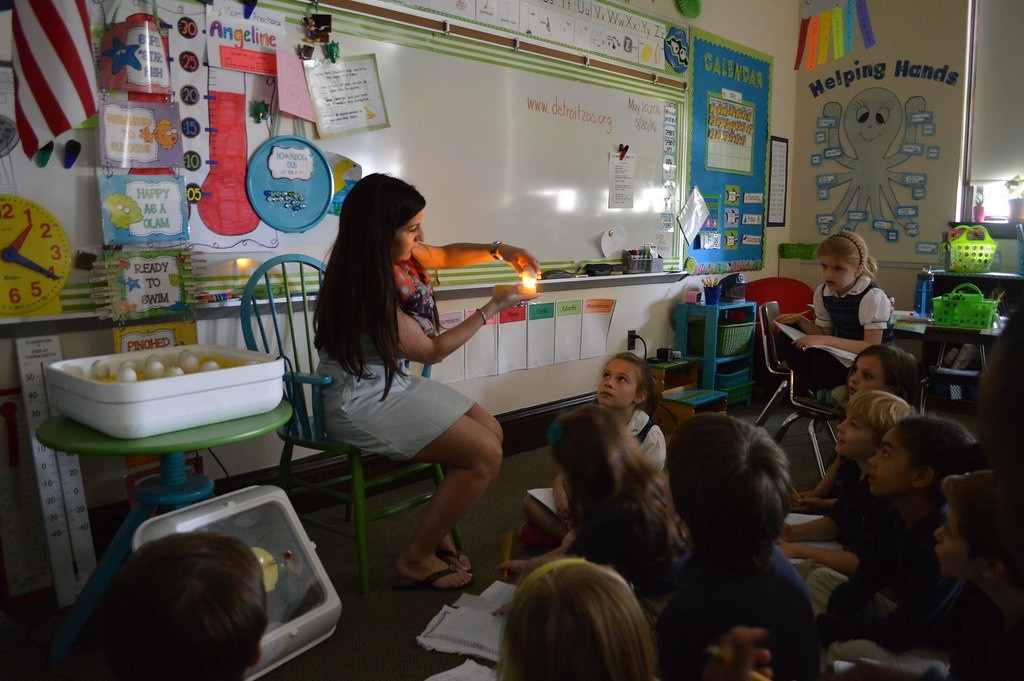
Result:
[629,249,648,259]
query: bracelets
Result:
[476,309,487,326]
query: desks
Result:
[33,403,293,669]
[879,309,1011,427]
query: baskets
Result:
[931,283,1001,329]
[688,318,757,356]
[939,224,998,273]
[697,366,750,388]
[716,380,756,403]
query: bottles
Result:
[915,264,934,319]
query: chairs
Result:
[754,300,800,428]
[771,369,842,480]
[240,251,465,595]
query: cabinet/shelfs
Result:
[646,358,729,431]
[674,300,757,398]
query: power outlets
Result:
[628,329,636,350]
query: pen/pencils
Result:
[778,308,813,323]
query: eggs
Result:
[90,349,219,383]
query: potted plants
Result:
[1006,175,1024,222]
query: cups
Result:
[705,286,721,304]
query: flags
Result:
[13,0,99,162]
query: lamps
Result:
[975,185,984,207]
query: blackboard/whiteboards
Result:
[1,0,688,336]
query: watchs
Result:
[490,241,502,260]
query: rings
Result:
[517,304,523,307]
[520,301,523,306]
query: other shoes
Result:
[828,386,848,408]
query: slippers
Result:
[397,564,474,589]
[434,549,472,573]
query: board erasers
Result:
[583,264,612,276]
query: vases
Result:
[972,206,985,223]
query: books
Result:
[775,320,858,368]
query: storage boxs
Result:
[45,344,284,441]
[715,366,755,404]
[622,250,663,274]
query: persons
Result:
[309,172,542,588]
[491,309,1024,681]
[92,531,268,681]
[773,229,894,400]
